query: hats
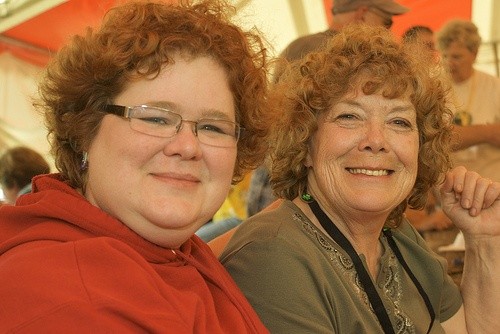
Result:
[331,0,411,15]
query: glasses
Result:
[101,102,246,149]
[368,6,393,30]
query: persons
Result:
[0,0,274,334]
[193,0,500,258]
[0,146,51,206]
[219,26,500,334]
